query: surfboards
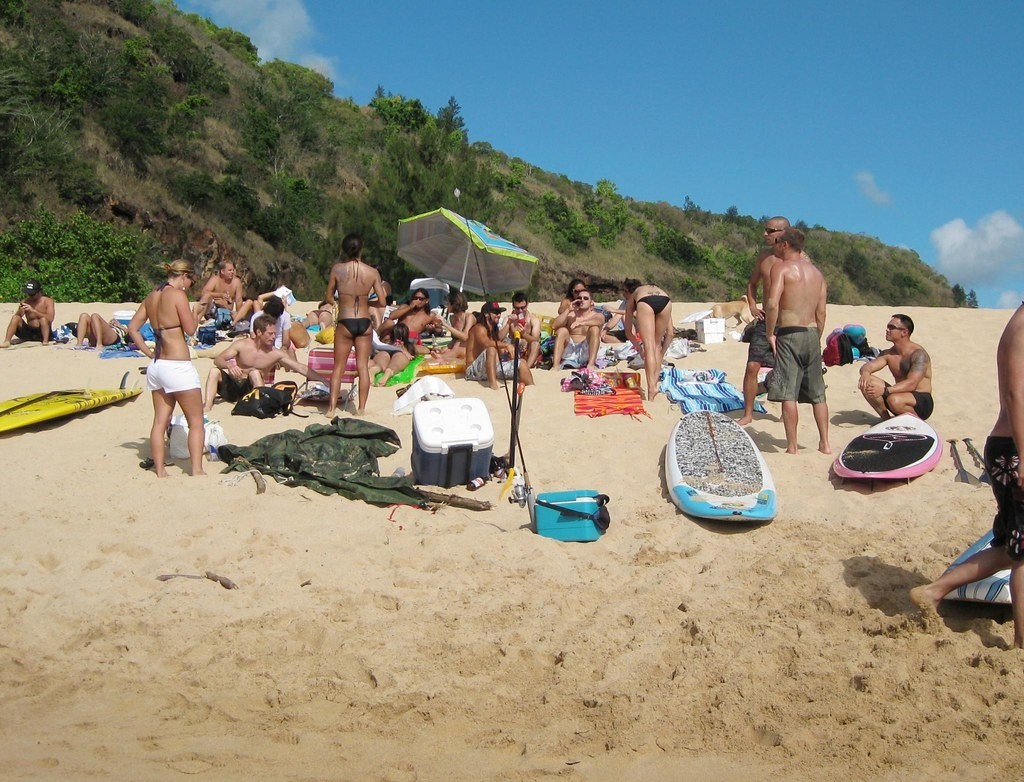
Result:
[665,409,778,522]
[833,411,943,491]
[0,371,145,433]
[940,528,1012,603]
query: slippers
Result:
[164,462,175,466]
[139,458,154,468]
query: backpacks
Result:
[822,325,873,367]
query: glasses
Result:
[765,227,783,234]
[775,239,778,244]
[577,296,589,300]
[413,296,426,301]
[182,272,196,287]
[573,288,579,295]
[887,324,904,331]
[492,310,500,314]
[515,305,527,310]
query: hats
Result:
[482,301,506,313]
[25,280,41,295]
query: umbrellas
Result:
[396,207,539,296]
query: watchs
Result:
[884,387,889,397]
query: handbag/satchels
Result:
[231,387,294,420]
[166,415,227,460]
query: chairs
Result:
[409,277,450,337]
[290,344,360,412]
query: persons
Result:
[858,314,934,422]
[325,234,387,419]
[766,226,831,455]
[128,259,211,479]
[0,281,55,349]
[76,313,128,351]
[736,217,812,427]
[195,259,674,413]
[910,302,1024,647]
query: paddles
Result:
[962,438,991,485]
[946,438,981,486]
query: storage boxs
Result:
[680,308,725,343]
[113,310,136,326]
[411,397,494,488]
[535,489,600,542]
